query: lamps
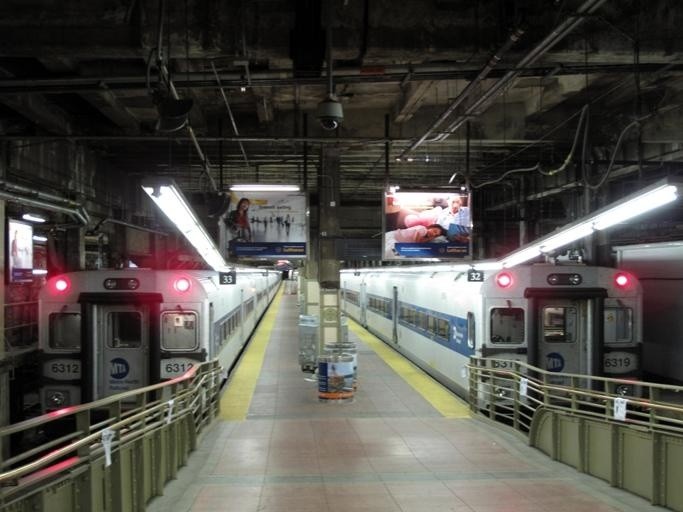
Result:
[470,175,683,274]
[139,176,283,275]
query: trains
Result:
[340,266,643,409]
[38,269,283,420]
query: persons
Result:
[224,197,254,240]
[10,229,23,266]
[250,214,296,236]
[385,223,447,257]
[436,194,470,231]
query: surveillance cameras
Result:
[315,99,344,131]
